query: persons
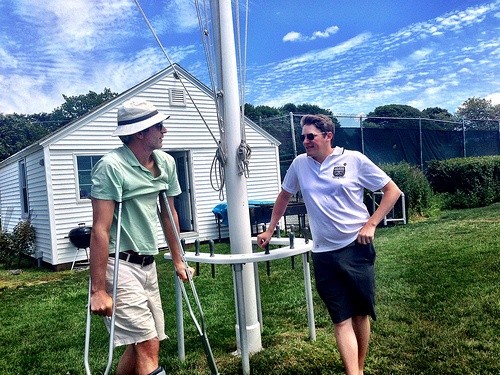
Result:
[90,101,195,375]
[257,114,403,375]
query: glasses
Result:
[299,132,327,142]
[154,122,163,130]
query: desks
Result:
[211,199,307,242]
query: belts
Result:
[109,252,154,268]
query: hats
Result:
[112,96,171,136]
